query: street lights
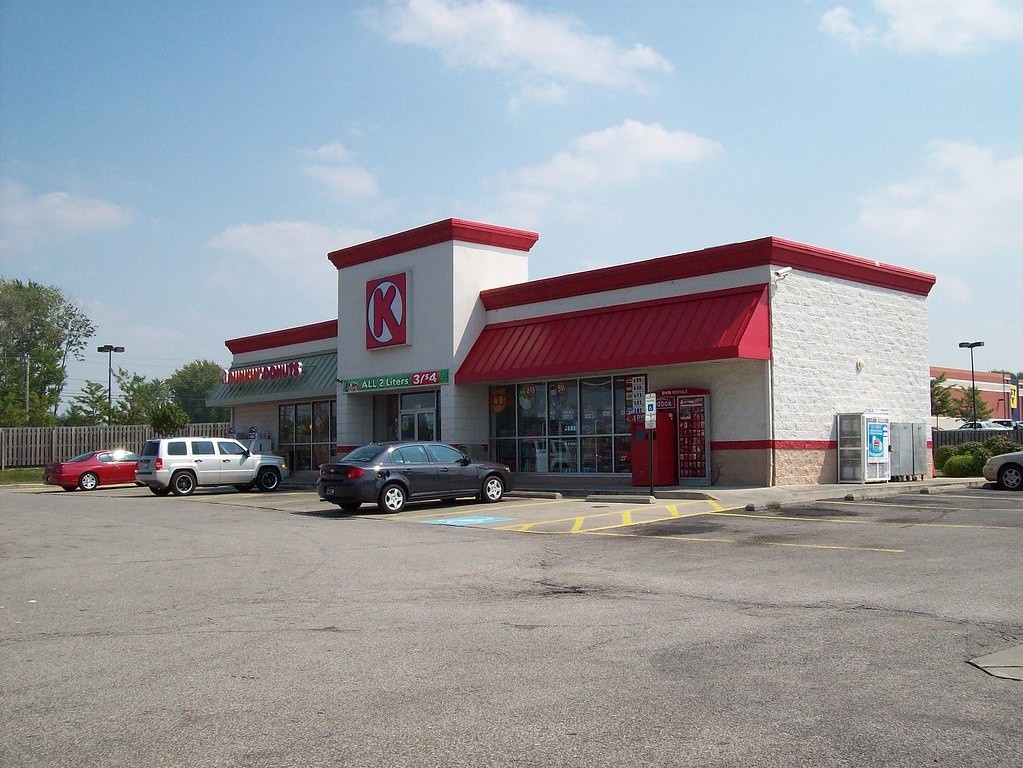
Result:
[958,342,985,429]
[97,344,125,425]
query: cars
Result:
[987,418,1023,431]
[43,449,143,491]
[522,435,634,475]
[316,440,516,514]
[957,421,1013,431]
[982,451,1023,490]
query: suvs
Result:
[135,435,289,497]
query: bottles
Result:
[561,421,577,448]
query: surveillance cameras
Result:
[774,267,792,276]
